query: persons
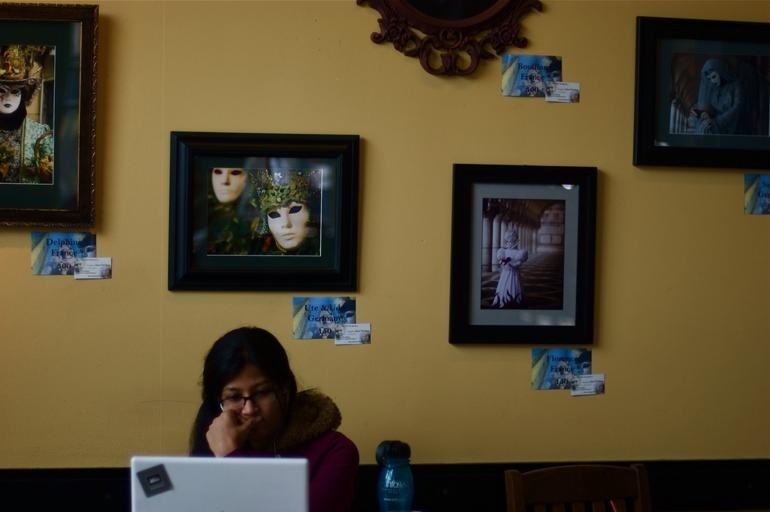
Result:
[209,167,263,254]
[188,326,358,511]
[249,169,320,255]
[699,60,742,134]
[0,46,52,184]
[491,229,528,309]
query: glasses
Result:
[217,386,279,413]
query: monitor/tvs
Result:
[131,457,310,512]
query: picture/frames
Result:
[0,1,100,228]
[448,157,595,346]
[166,130,362,292]
[630,13,769,172]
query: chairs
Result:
[503,462,655,510]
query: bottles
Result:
[377,438,415,512]
